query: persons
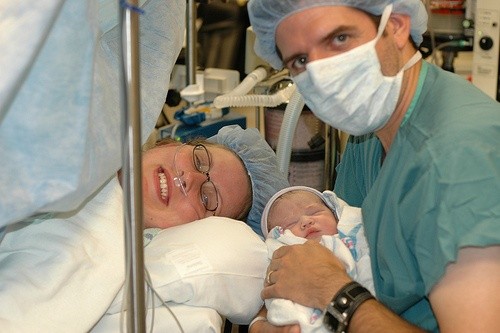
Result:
[260,183,377,333]
[129,124,287,233]
[247,0,500,333]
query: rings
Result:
[267,269,276,284]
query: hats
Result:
[260,185,327,239]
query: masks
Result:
[294,6,422,135]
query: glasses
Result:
[193,142,219,218]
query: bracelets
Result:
[247,315,266,331]
[323,282,372,333]
